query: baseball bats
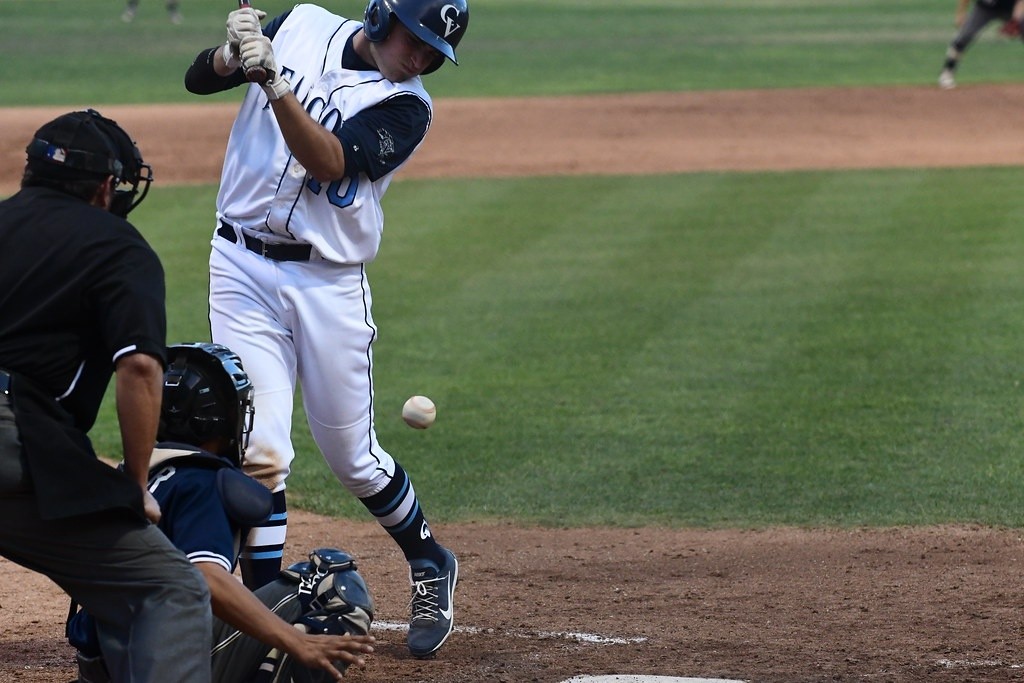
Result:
[237,0,269,83]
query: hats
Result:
[26,114,119,183]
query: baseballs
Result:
[402,394,437,430]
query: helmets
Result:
[363,0,469,75]
[155,342,254,469]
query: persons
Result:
[184,0,470,660]
[120,0,184,25]
[937,0,1024,91]
[64,341,377,683]
[0,108,214,683]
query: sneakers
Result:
[404,543,458,660]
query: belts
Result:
[217,219,324,261]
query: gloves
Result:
[223,8,267,69]
[239,36,290,100]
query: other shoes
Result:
[939,67,956,91]
